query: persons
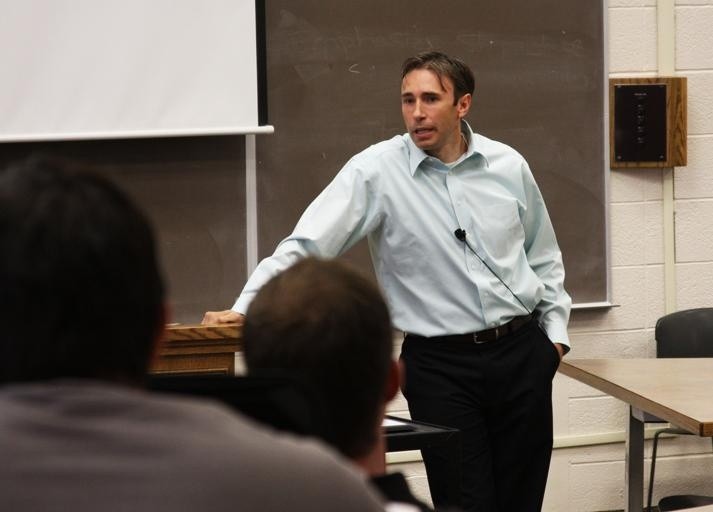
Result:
[243,257,434,512]
[0,154,383,512]
[201,50,573,512]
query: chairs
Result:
[647,306,713,512]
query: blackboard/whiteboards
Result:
[0,0,621,326]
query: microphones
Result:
[452,225,469,244]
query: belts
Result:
[453,318,524,344]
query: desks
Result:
[548,352,713,512]
